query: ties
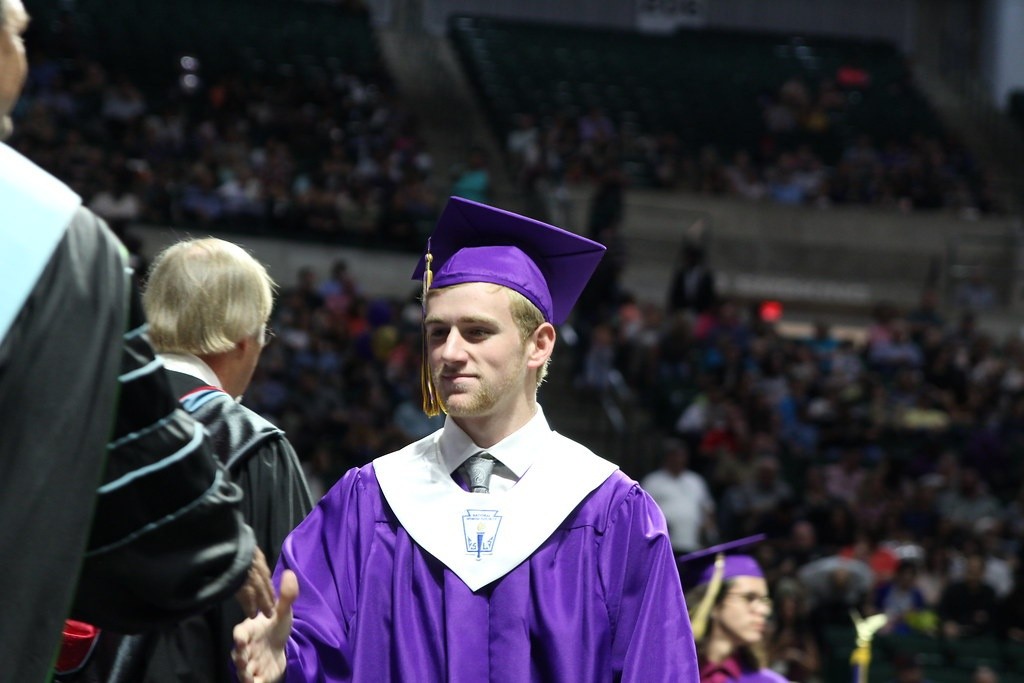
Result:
[456,451,499,494]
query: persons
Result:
[6,52,978,225]
[0,0,315,683]
[231,197,700,683]
[243,231,1024,683]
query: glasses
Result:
[726,591,772,607]
[262,330,273,347]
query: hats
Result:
[410,195,606,417]
[678,534,766,640]
